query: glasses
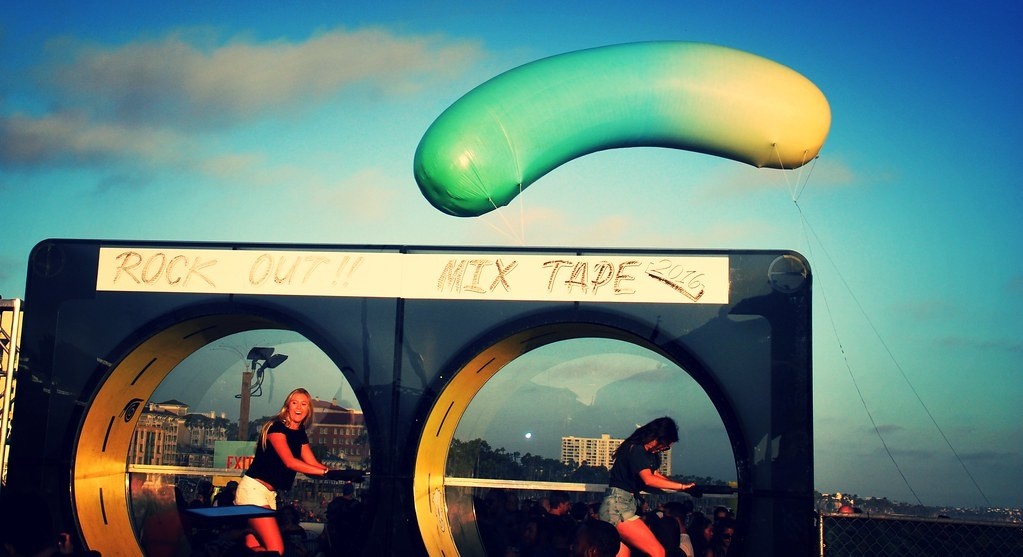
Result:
[722,533,732,538]
[662,442,671,452]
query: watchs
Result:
[323,468,329,476]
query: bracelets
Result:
[681,484,684,491]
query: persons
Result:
[232,384,347,556]
[596,414,700,557]
[172,474,737,557]
